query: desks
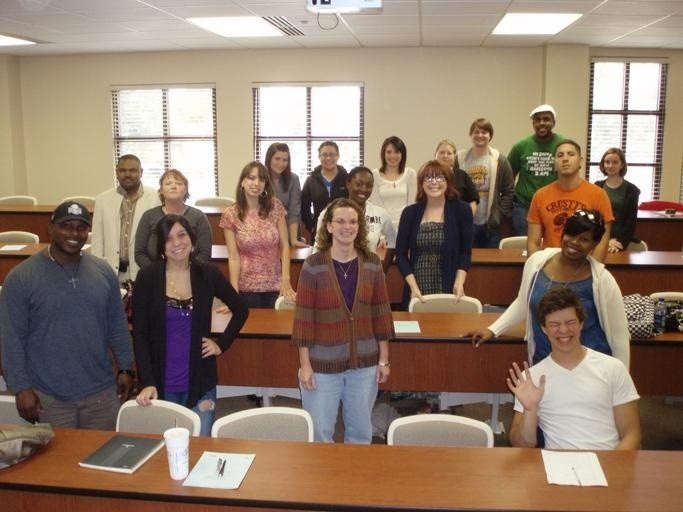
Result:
[1,206,683,251]
[130,307,683,435]
[0,425,683,511]
[0,243,683,305]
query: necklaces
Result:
[47,244,84,291]
[164,263,193,316]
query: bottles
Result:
[654,298,666,334]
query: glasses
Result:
[423,174,446,182]
[319,153,338,157]
[575,209,601,225]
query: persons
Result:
[458,208,631,448]
[90,155,212,283]
[131,215,249,437]
[219,102,642,308]
[0,200,137,429]
[505,287,642,451]
[290,198,396,445]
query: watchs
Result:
[118,370,136,379]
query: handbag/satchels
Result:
[623,293,654,339]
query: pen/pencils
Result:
[217,458,222,475]
[218,459,226,477]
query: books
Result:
[78,434,165,474]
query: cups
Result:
[163,428,189,480]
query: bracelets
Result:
[378,362,390,367]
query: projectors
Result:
[306,1,382,14]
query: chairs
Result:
[274,295,297,308]
[639,201,683,210]
[0,231,41,246]
[195,198,235,206]
[627,242,646,251]
[212,407,314,442]
[410,295,481,310]
[62,196,96,211]
[115,400,202,437]
[1,196,38,207]
[386,414,494,448]
[1,395,39,424]
[500,236,542,249]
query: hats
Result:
[530,104,556,119]
[51,202,92,228]
